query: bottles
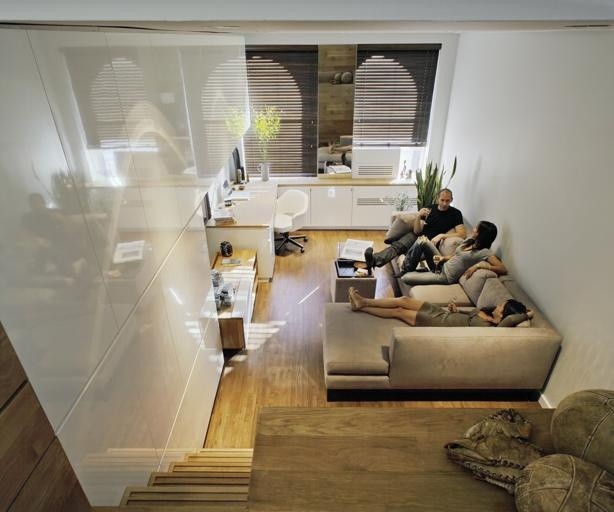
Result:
[220,241,234,257]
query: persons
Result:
[348,285,534,328]
[27,234,78,337]
[390,220,507,284]
[329,145,352,155]
[22,192,91,268]
[364,187,468,272]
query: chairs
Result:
[274,190,309,255]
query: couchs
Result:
[322,210,564,401]
[318,135,353,170]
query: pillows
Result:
[384,213,416,244]
[438,229,465,256]
[476,278,531,327]
[458,266,497,307]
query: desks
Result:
[210,249,259,351]
[206,183,278,283]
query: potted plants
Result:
[252,105,280,180]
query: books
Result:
[215,203,237,225]
[230,190,253,201]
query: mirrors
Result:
[317,45,357,178]
